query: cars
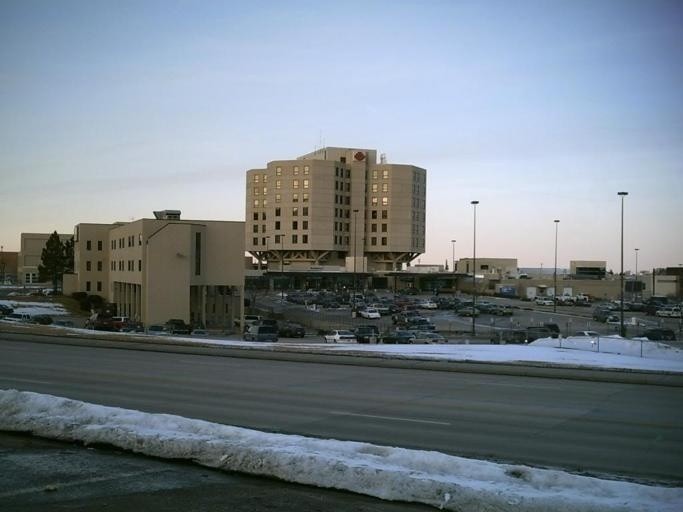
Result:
[0,299,209,339]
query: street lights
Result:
[352,209,359,310]
[635,248,639,279]
[552,220,560,310]
[451,240,455,271]
[279,234,285,298]
[616,192,627,334]
[470,201,479,333]
